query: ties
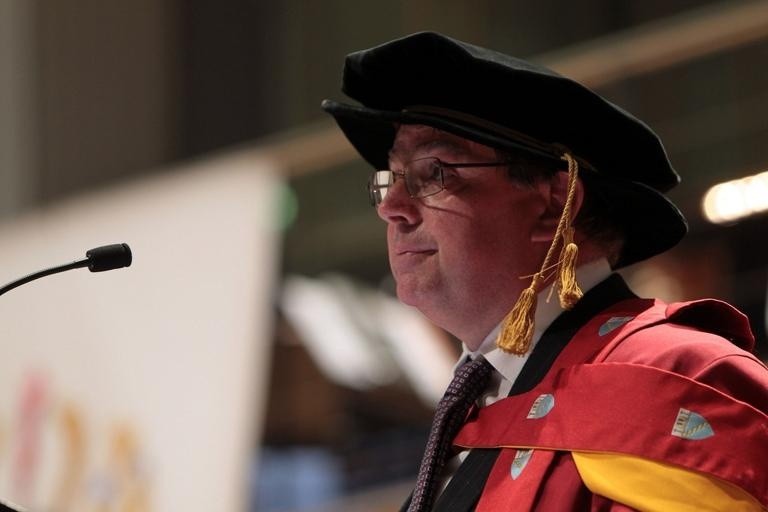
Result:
[407,352,496,511]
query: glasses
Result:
[364,155,509,209]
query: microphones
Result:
[0,243,132,510]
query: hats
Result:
[320,30,689,357]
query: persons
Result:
[320,31,768,512]
